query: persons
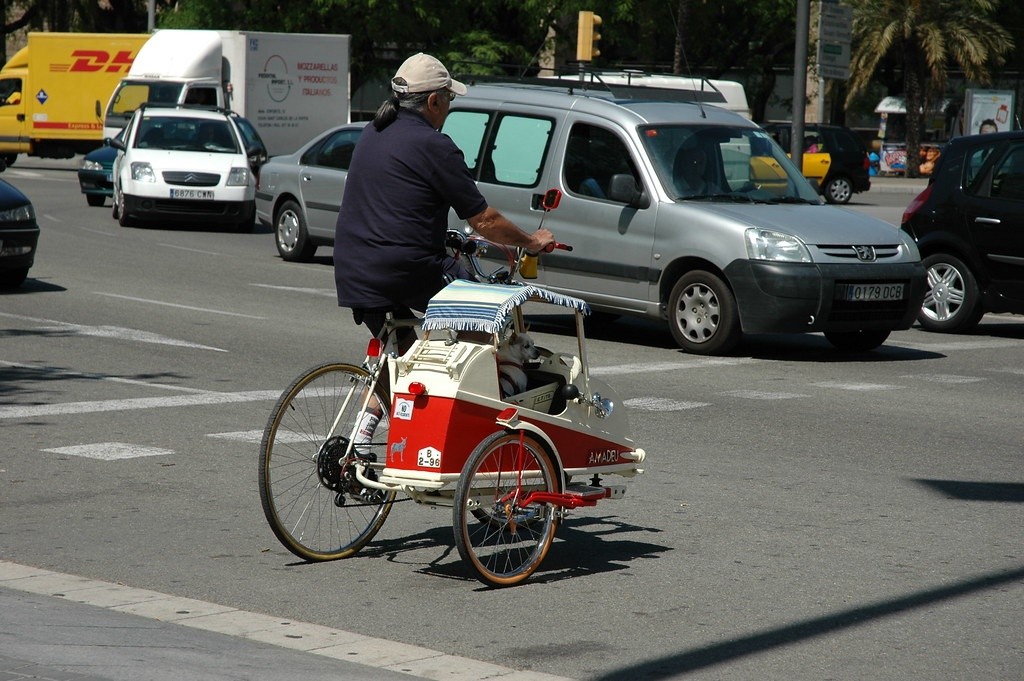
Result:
[674,150,755,195]
[199,125,214,142]
[805,136,817,153]
[979,119,997,134]
[577,148,617,198]
[6,85,21,104]
[333,52,556,454]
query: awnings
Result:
[874,96,951,112]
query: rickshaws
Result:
[254,187,648,589]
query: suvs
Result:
[747,118,872,205]
[431,80,930,358]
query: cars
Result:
[253,119,372,263]
[77,102,271,208]
[104,100,259,233]
[897,129,1024,334]
[0,176,40,291]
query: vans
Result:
[532,67,754,195]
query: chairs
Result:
[566,142,628,198]
[671,147,707,195]
[140,127,167,148]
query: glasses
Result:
[425,90,456,101]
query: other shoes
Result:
[351,465,384,503]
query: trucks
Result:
[0,31,156,168]
[100,26,354,186]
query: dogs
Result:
[496,330,541,401]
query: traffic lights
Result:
[575,10,602,63]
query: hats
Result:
[391,52,468,96]
